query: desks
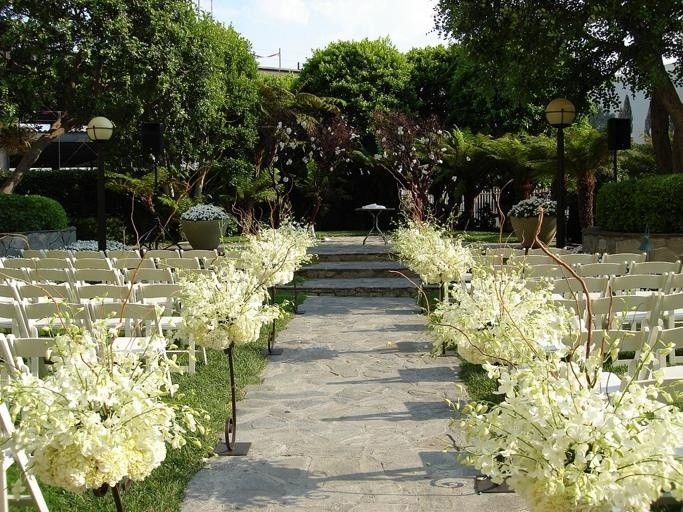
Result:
[356,207,395,245]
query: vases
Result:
[180,219,225,251]
[508,215,557,248]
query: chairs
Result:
[0,248,249,512]
[462,248,682,405]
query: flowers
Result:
[425,266,682,508]
[166,258,297,354]
[222,205,322,282]
[177,201,229,221]
[1,285,217,495]
[505,194,558,217]
[379,196,476,285]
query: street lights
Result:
[84,114,115,258]
[541,95,577,248]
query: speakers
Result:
[607,118,631,150]
